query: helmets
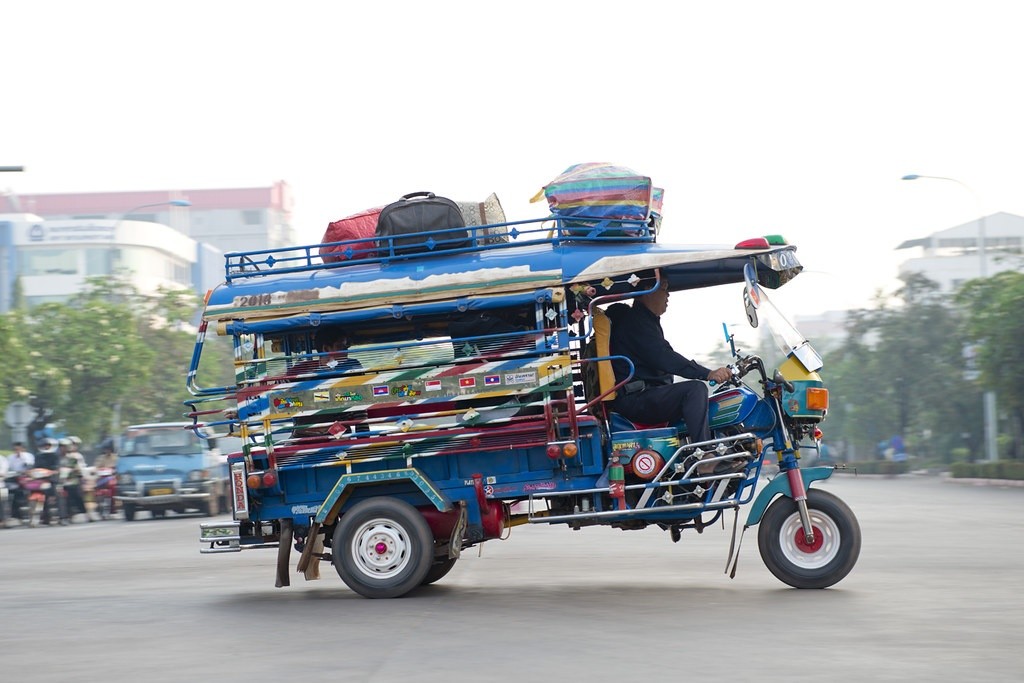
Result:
[37,439,58,454]
[70,436,82,443]
[58,438,71,445]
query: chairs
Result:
[590,310,671,432]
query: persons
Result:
[877,433,906,462]
[807,441,835,470]
[585,271,750,476]
[223,327,374,455]
[0,433,119,528]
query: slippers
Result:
[699,459,748,476]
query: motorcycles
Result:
[181,214,863,599]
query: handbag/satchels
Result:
[319,163,665,266]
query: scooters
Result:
[0,464,119,525]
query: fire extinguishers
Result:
[609,446,630,497]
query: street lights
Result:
[902,174,998,461]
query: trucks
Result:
[118,421,232,521]
